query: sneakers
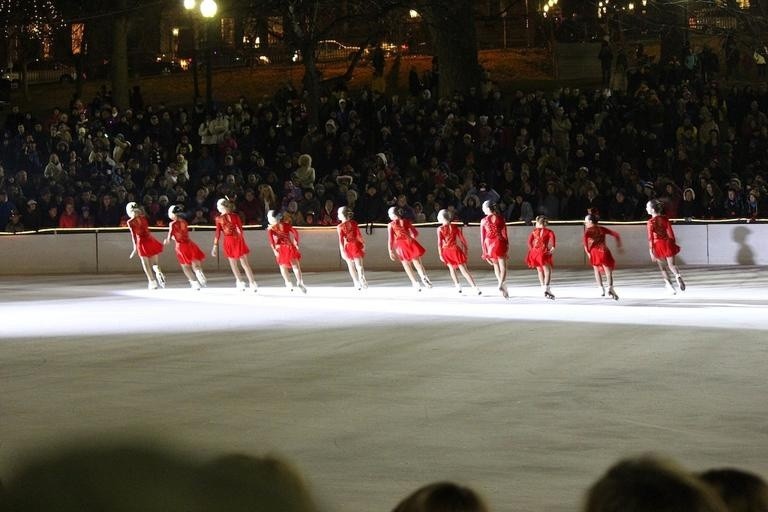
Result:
[676,274,686,292]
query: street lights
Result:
[183,0,200,104]
[201,1,217,111]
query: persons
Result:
[478,199,512,301]
[336,205,369,292]
[586,454,768,512]
[645,199,686,295]
[385,206,434,292]
[0,23,768,236]
[524,214,555,300]
[392,482,485,512]
[210,198,259,293]
[581,213,624,301]
[266,209,307,293]
[163,205,207,290]
[125,201,166,291]
[435,208,483,296]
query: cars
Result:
[2,59,75,84]
[195,44,271,72]
[299,39,366,65]
[97,57,180,79]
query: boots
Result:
[413,275,433,293]
[197,270,208,288]
[156,271,167,289]
[353,278,368,291]
[296,281,307,294]
[236,282,245,294]
[456,283,483,294]
[544,284,556,301]
[498,280,510,300]
[607,285,620,301]
[286,281,293,293]
[250,281,258,293]
[147,280,158,291]
[190,281,201,292]
